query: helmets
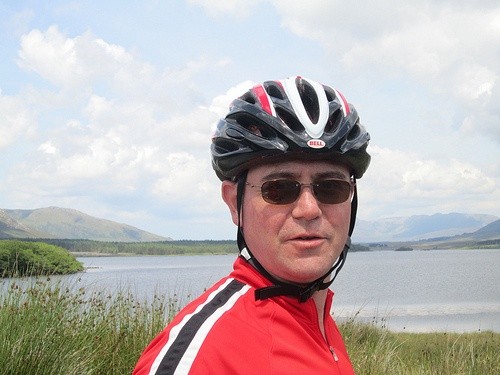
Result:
[210,76,371,181]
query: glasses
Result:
[244,177,355,206]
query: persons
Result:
[127,74,371,375]
